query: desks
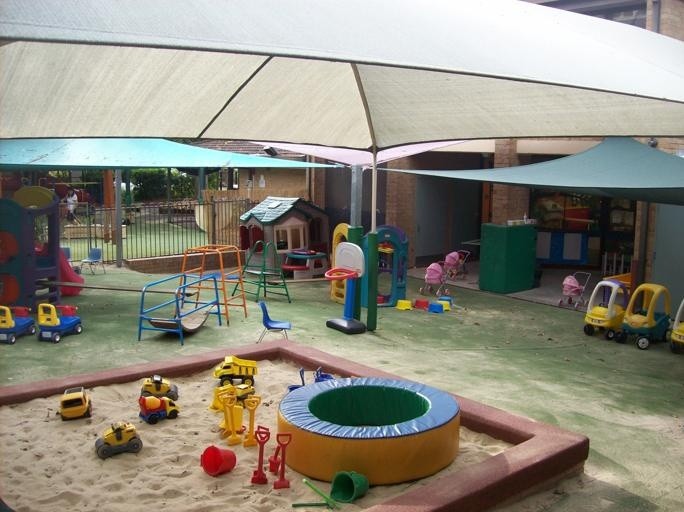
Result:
[285,250,329,279]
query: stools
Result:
[280,263,311,281]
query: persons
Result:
[62,187,83,227]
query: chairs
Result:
[60,246,74,266]
[80,247,106,276]
